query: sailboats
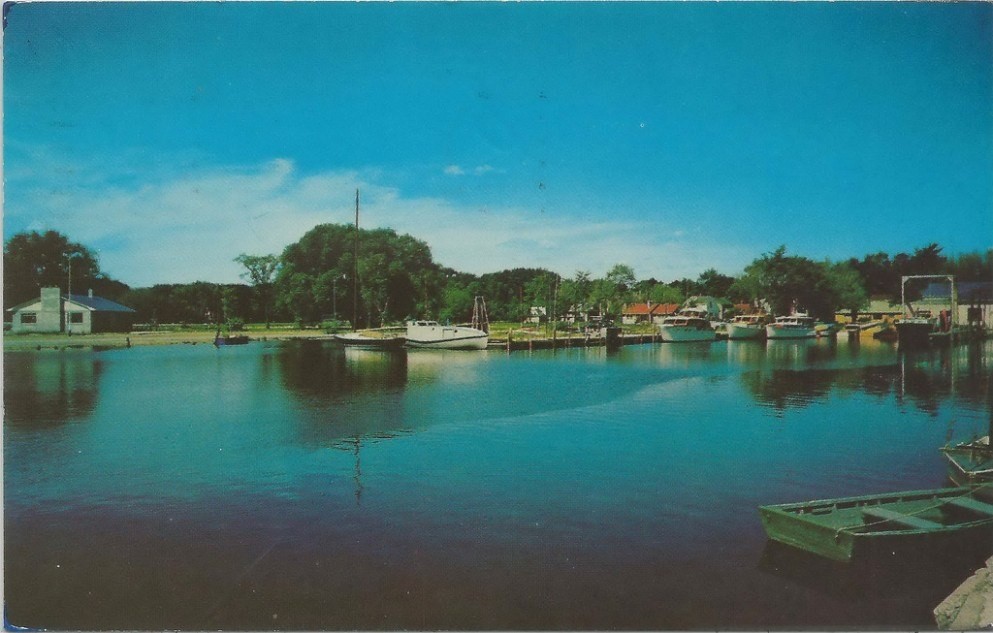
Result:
[334,188,406,348]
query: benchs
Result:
[862,508,941,529]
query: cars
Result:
[581,319,616,332]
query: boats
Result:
[846,308,993,344]
[658,309,717,342]
[939,433,993,488]
[213,333,251,345]
[813,322,835,337]
[404,320,488,350]
[727,307,775,340]
[714,323,729,340]
[766,312,820,339]
[756,480,993,571]
